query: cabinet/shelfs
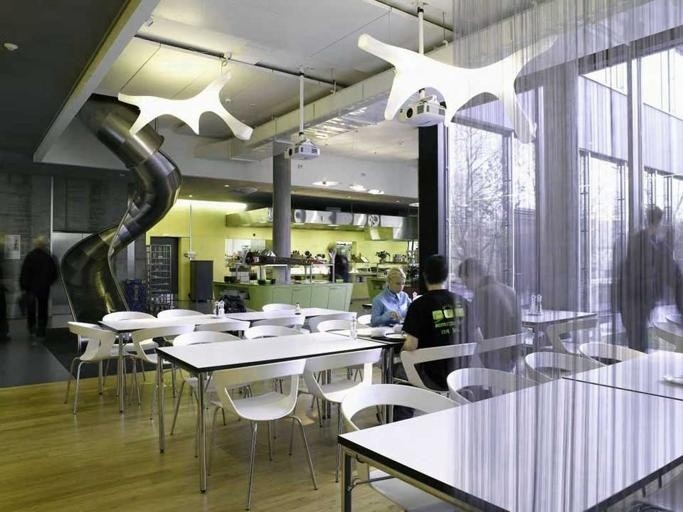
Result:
[190,260,213,301]
[312,285,346,311]
[145,244,172,318]
[272,287,311,307]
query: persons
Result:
[0,249,12,341]
[370,268,414,422]
[612,207,663,352]
[458,257,522,402]
[377,254,469,423]
[19,237,58,342]
[329,248,352,305]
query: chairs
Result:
[578,342,649,363]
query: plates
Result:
[385,333,410,339]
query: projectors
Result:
[398,95,445,126]
[284,139,320,160]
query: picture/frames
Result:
[3,234,21,260]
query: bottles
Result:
[294,301,301,315]
[536,294,542,314]
[530,294,536,315]
